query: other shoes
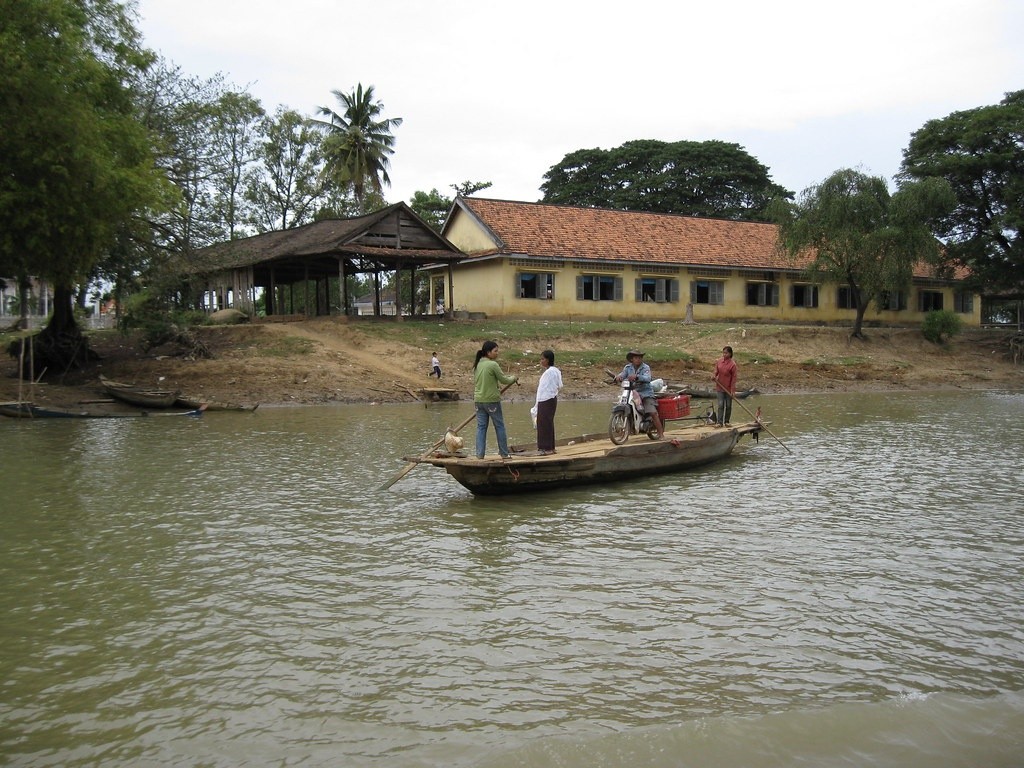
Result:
[715,424,722,428]
[534,448,556,456]
[502,454,512,459]
[725,423,732,428]
[428,373,430,378]
[439,377,443,380]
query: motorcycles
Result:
[602,370,665,446]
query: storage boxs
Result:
[658,396,690,419]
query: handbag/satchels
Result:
[530,404,538,430]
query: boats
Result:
[667,383,751,400]
[0,377,259,418]
[402,406,773,497]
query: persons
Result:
[616,349,666,441]
[472,341,519,459]
[709,346,738,428]
[427,352,443,379]
[534,351,563,457]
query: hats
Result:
[626,349,646,363]
